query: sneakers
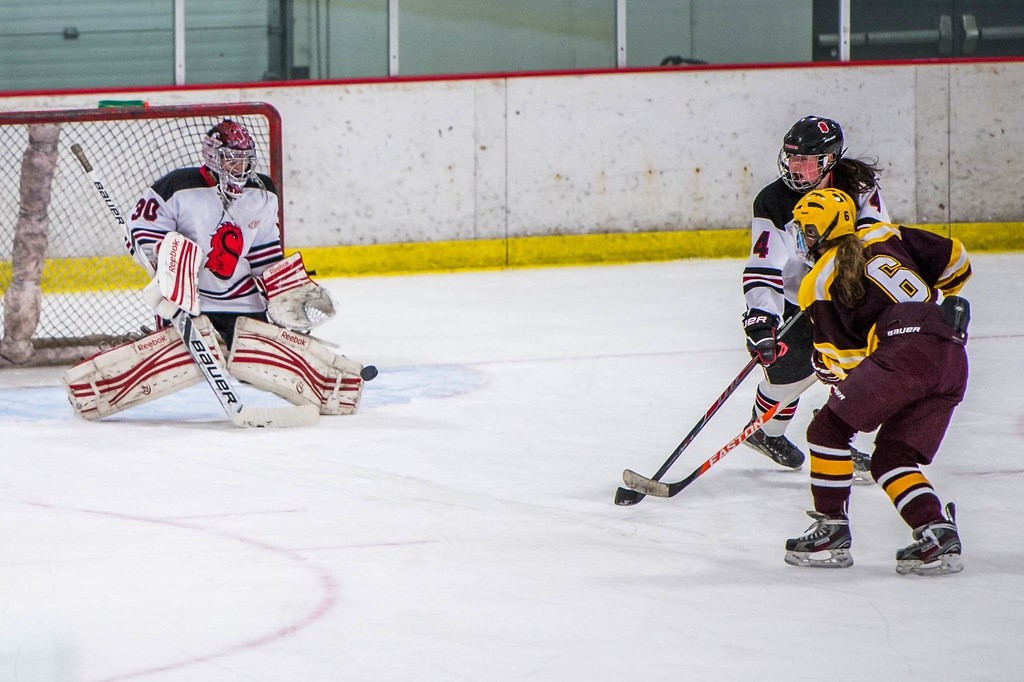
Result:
[895,502,963,577]
[742,421,806,471]
[784,511,854,568]
[850,445,874,486]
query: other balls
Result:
[359,365,379,382]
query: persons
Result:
[784,188,972,577]
[742,114,892,486]
[63,119,364,420]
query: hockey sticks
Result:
[615,306,805,505]
[624,374,820,498]
[70,141,320,430]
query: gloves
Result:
[742,308,788,366]
[811,350,841,386]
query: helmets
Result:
[202,119,258,187]
[792,188,856,260]
[777,115,846,191]
